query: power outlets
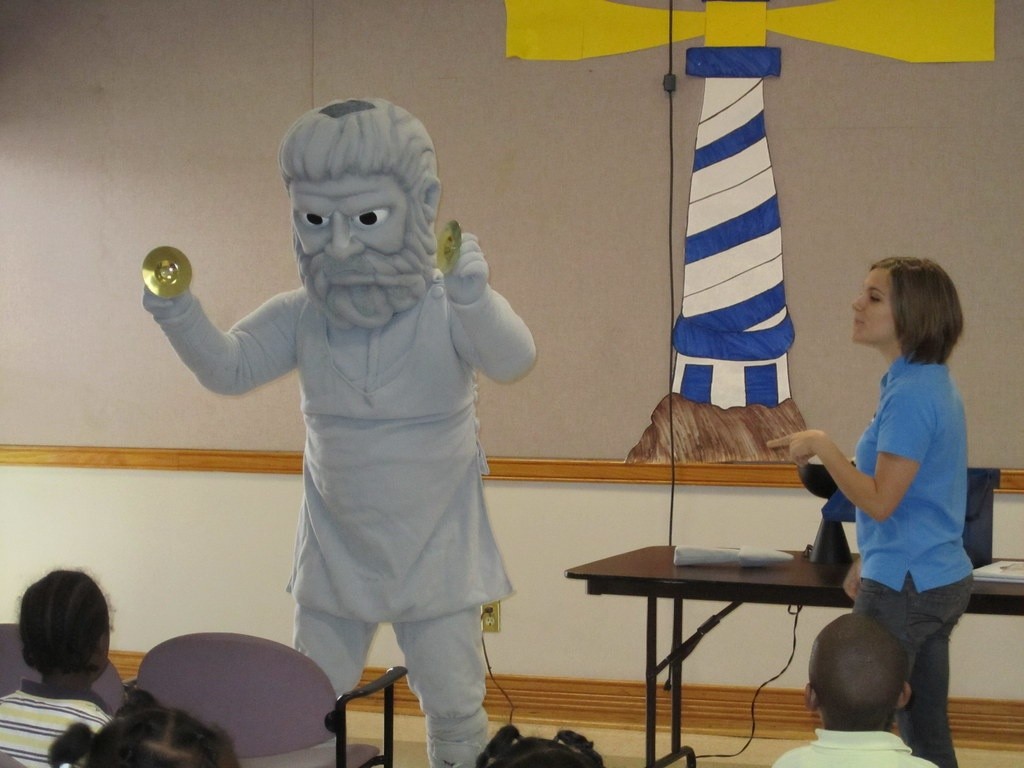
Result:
[481,602,501,633]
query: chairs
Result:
[0,623,408,768]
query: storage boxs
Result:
[821,467,1000,568]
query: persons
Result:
[0,569,119,767]
[765,258,977,768]
[770,612,944,767]
[138,97,535,768]
[48,707,241,768]
[475,724,606,768]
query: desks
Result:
[564,546,1024,768]
